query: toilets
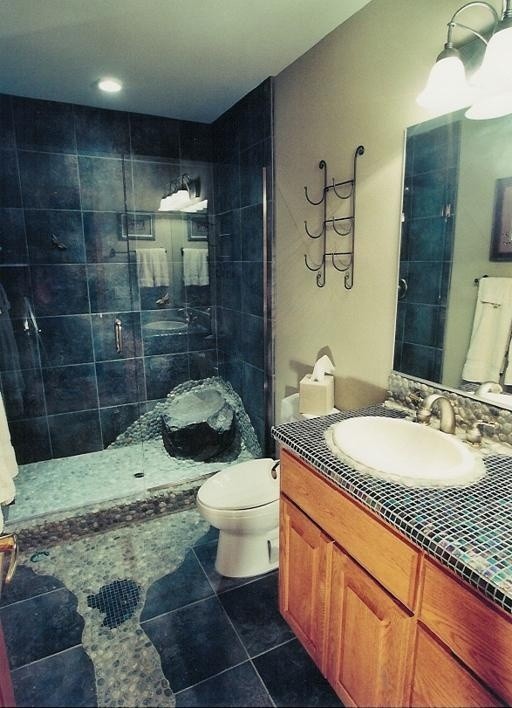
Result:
[196,394,343,578]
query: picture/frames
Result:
[117,210,156,241]
[489,178,512,261]
[187,215,210,241]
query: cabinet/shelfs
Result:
[278,447,421,708]
[408,554,512,708]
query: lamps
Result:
[159,174,208,213]
[416,0,512,120]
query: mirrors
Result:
[391,108,512,414]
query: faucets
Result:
[473,380,502,398]
[416,394,456,434]
[178,308,188,318]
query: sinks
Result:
[467,392,512,411]
[325,416,486,490]
[143,321,187,331]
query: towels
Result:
[154,248,170,287]
[504,338,512,385]
[0,393,19,534]
[0,285,26,395]
[135,248,154,288]
[462,276,512,384]
[183,249,209,286]
